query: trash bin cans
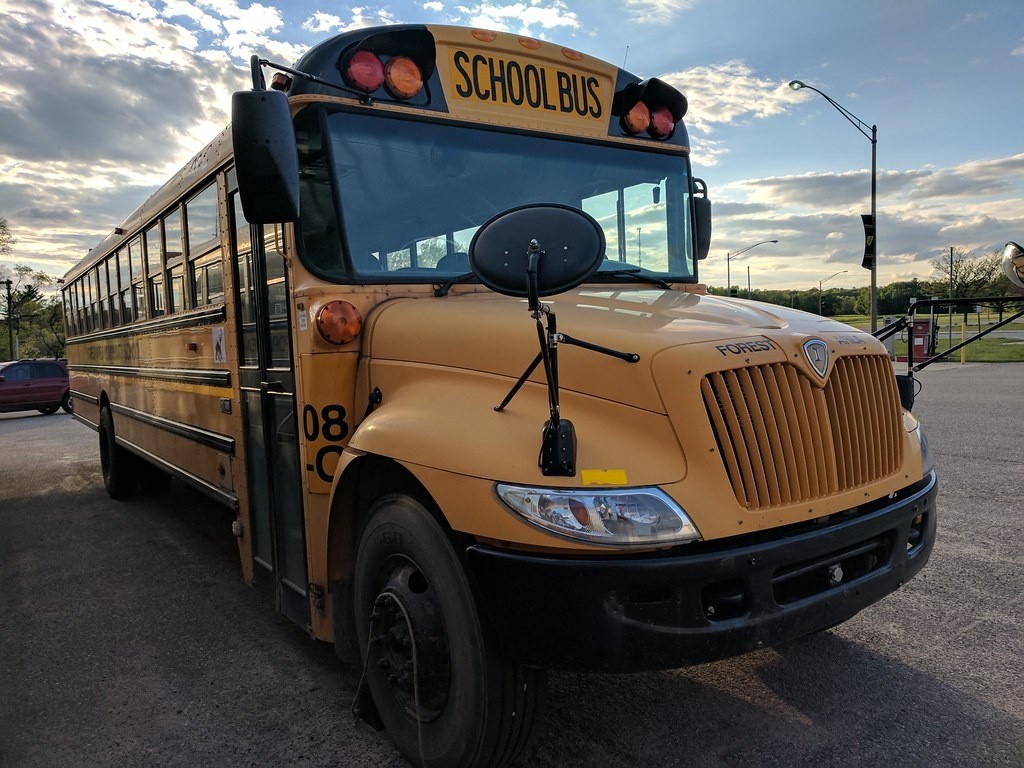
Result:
[912,318,936,357]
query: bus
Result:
[58,22,1024,768]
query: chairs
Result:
[359,247,471,272]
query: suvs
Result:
[0,356,72,415]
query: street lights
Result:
[637,227,642,267]
[789,78,879,336]
[818,270,849,317]
[727,239,779,298]
